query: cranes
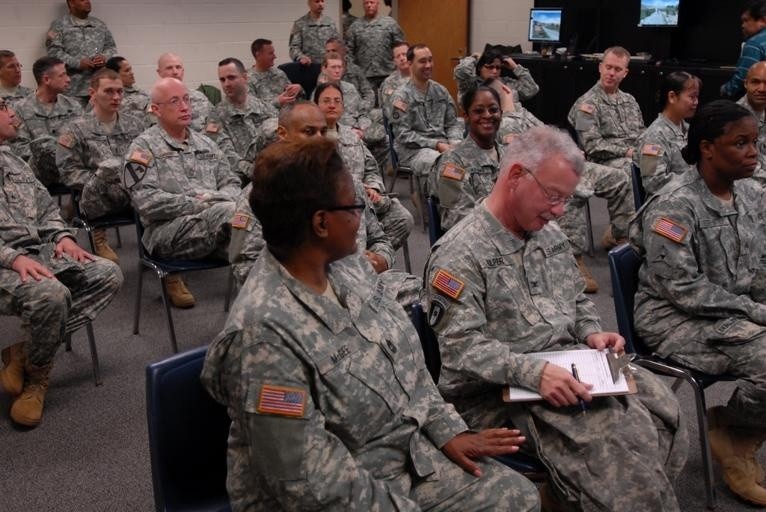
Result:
[502,345,640,404]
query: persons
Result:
[311,52,390,179]
[85,55,150,124]
[313,81,415,252]
[45,0,117,110]
[289,0,342,65]
[245,39,306,111]
[0,96,124,427]
[199,135,533,512]
[567,47,648,255]
[0,49,36,103]
[633,100,766,504]
[716,1,766,103]
[388,44,465,227]
[121,77,242,264]
[317,37,383,126]
[453,48,539,109]
[735,62,766,181]
[343,1,407,109]
[56,67,195,308]
[427,86,508,232]
[637,71,702,203]
[423,125,689,512]
[204,57,280,184]
[478,76,641,293]
[144,52,215,132]
[10,56,121,266]
[229,99,424,315]
[378,40,411,122]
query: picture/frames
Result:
[501,53,747,125]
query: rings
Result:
[570,363,588,418]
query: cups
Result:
[526,165,574,207]
[319,99,344,105]
[483,64,502,71]
[326,196,366,212]
[0,102,7,109]
[8,63,23,69]
[157,96,191,106]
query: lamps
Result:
[66,317,105,388]
[146,342,234,512]
[70,183,140,258]
[134,216,244,354]
[195,83,222,106]
[426,193,443,246]
[402,239,412,276]
[404,242,569,510]
[608,241,743,511]
[389,122,428,232]
[630,162,645,212]
[50,184,71,208]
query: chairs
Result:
[707,386,766,506]
[164,274,196,307]
[9,358,54,426]
[0,340,38,395]
[95,229,120,264]
[575,253,599,292]
[602,224,639,254]
[411,191,429,225]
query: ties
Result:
[638,0,680,31]
[528,8,563,46]
